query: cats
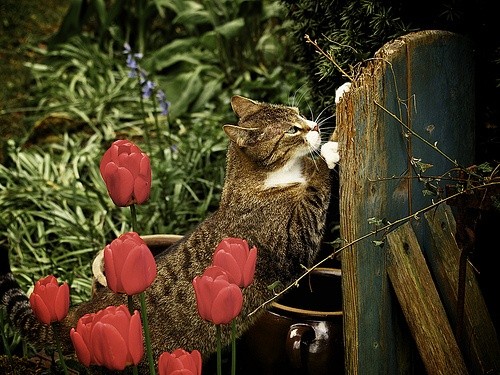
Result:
[0,80,351,375]
